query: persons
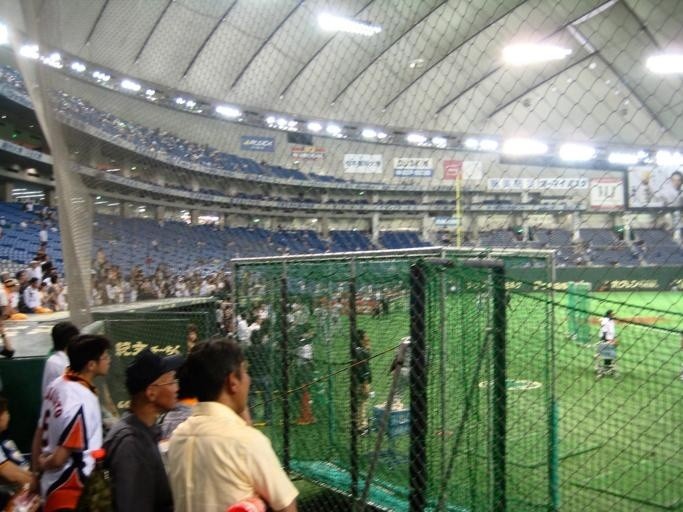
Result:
[643,170,682,208]
[0,195,391,509]
[1,63,250,172]
[595,309,622,374]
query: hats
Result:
[399,336,411,344]
[128,356,183,395]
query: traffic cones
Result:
[294,392,317,425]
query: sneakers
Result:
[358,427,369,436]
[295,416,316,426]
[263,415,274,427]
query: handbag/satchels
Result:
[599,342,616,359]
